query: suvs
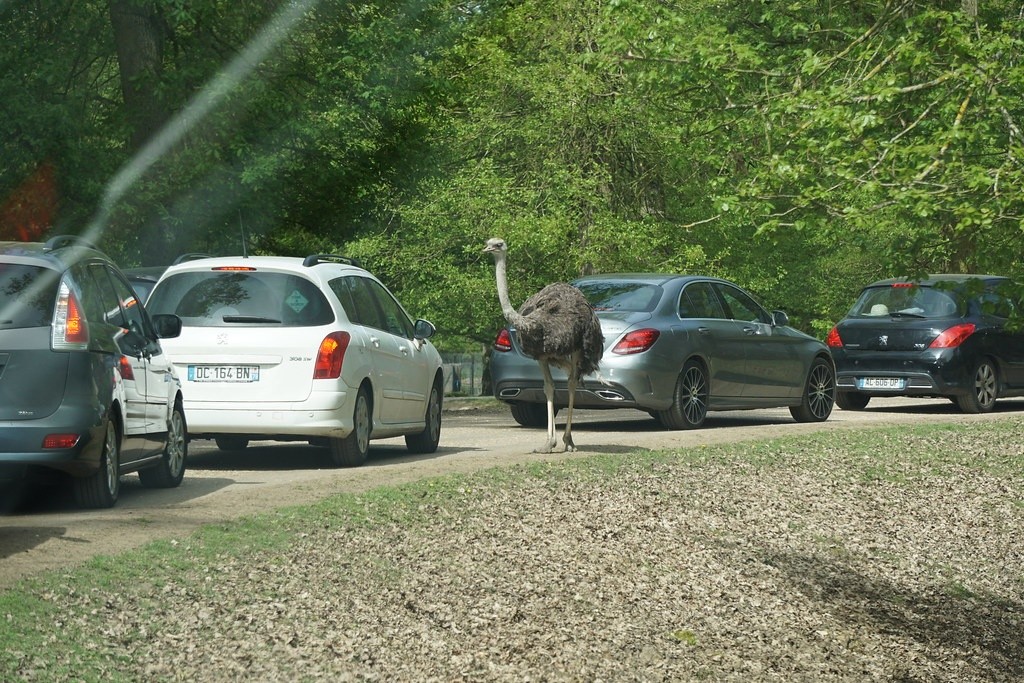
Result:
[0,236,188,511]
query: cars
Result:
[143,252,447,467]
[488,272,838,430]
[826,272,1024,413]
[119,267,166,304]
